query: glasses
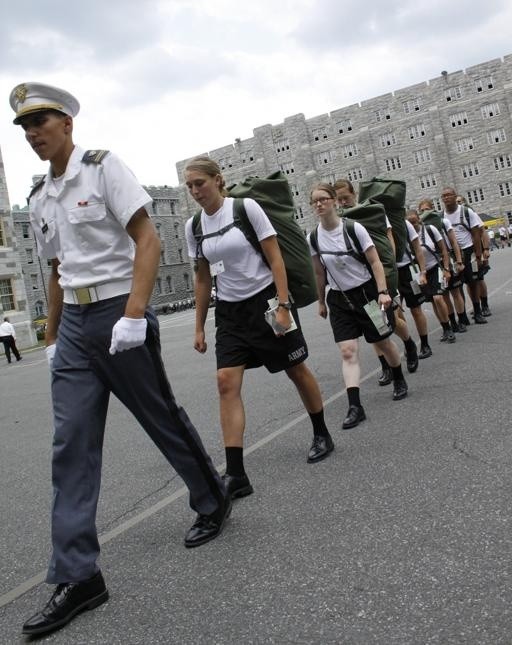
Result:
[309,195,334,206]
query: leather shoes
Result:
[458,323,467,333]
[447,330,457,343]
[452,325,459,332]
[20,566,112,639]
[305,433,335,464]
[405,345,419,374]
[181,488,235,552]
[471,311,488,325]
[217,471,254,502]
[375,368,393,385]
[465,317,470,325]
[439,330,448,341]
[481,306,491,317]
[391,377,409,402]
[339,405,367,430]
[418,344,433,359]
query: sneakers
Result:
[6,356,23,364]
[490,242,510,252]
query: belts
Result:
[58,277,137,306]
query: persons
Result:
[0,315,20,363]
[306,185,412,427]
[332,176,418,387]
[397,187,511,359]
[175,151,336,497]
[10,80,233,639]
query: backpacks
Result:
[310,202,398,299]
[418,209,446,256]
[356,178,409,265]
[192,168,322,311]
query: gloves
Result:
[108,315,149,357]
[43,345,58,368]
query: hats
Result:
[8,80,82,127]
